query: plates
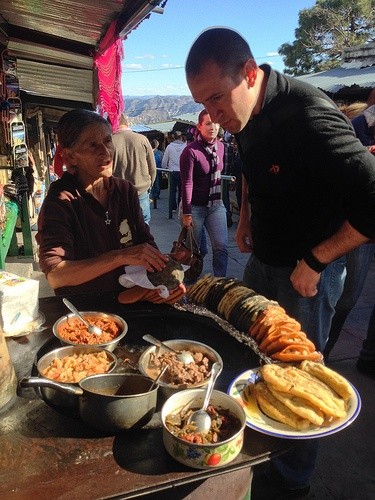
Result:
[228,363,362,440]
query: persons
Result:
[187,25,375,360]
[35,108,188,308]
[130,116,249,230]
[317,103,375,374]
[99,111,158,226]
[178,109,233,280]
[338,100,368,121]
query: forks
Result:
[187,361,223,433]
[142,334,196,365]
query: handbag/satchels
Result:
[171,225,201,266]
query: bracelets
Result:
[302,249,329,274]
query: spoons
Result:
[63,298,103,336]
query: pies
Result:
[147,258,352,430]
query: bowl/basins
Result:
[52,311,129,353]
[36,344,117,417]
[161,387,247,470]
[138,339,223,399]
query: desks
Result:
[0,283,326,500]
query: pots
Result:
[20,372,159,431]
